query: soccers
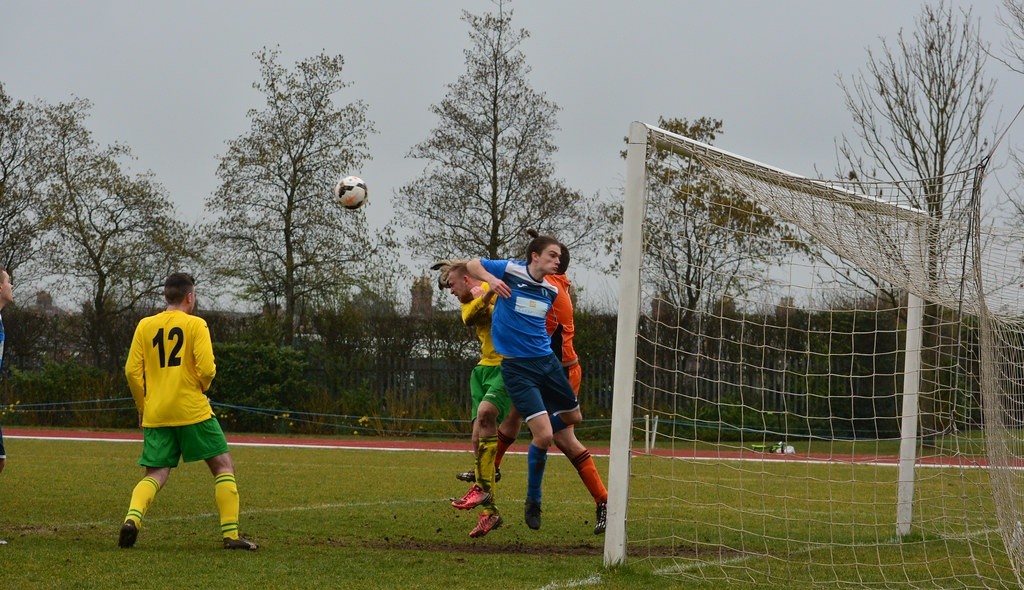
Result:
[334,175,369,210]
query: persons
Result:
[119,274,259,551]
[446,263,517,537]
[465,236,582,530]
[456,242,608,534]
[0,268,15,545]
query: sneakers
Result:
[593,502,607,534]
[456,466,501,483]
[118,519,139,548]
[451,485,494,510]
[223,532,259,551]
[524,498,543,530]
[470,512,503,538]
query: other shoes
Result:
[0,540,8,545]
